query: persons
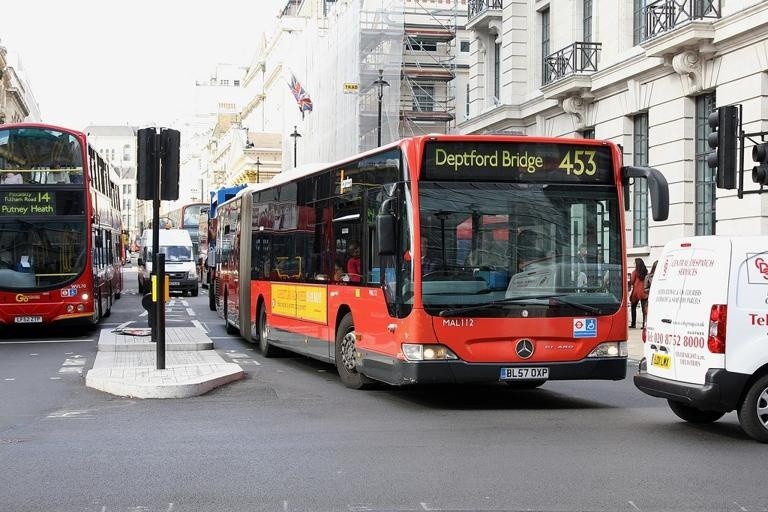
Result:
[0,237,82,285]
[627,258,648,329]
[0,159,24,185]
[45,159,71,185]
[246,201,300,230]
[642,261,659,295]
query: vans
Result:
[632,234,768,443]
[137,228,199,297]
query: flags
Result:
[285,71,314,118]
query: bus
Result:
[160,201,210,270]
[206,133,669,387]
[1,121,125,326]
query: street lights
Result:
[253,156,264,183]
[372,68,391,148]
[289,125,302,168]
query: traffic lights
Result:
[707,102,738,191]
[751,136,766,188]
